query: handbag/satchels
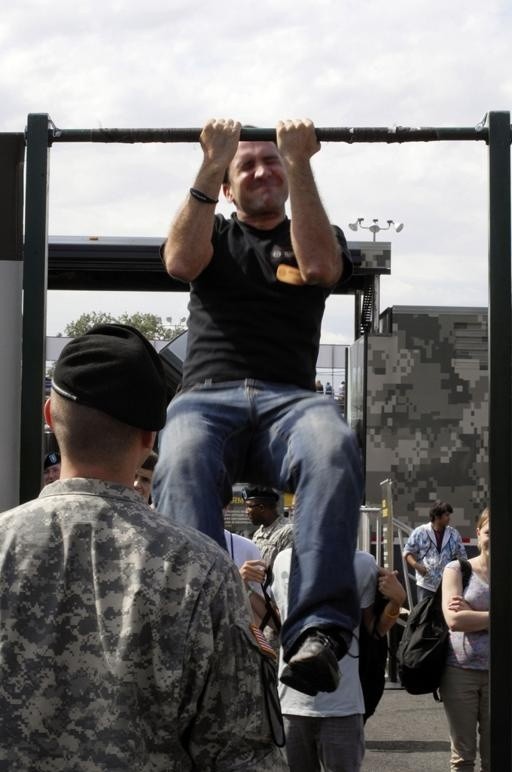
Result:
[396,595,450,694]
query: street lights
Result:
[348,217,404,242]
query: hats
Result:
[50,323,168,432]
[43,450,62,471]
[240,485,280,501]
[141,451,157,469]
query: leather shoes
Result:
[280,625,359,696]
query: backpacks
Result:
[261,566,387,725]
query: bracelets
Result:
[188,186,219,208]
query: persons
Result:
[150,118,367,696]
[220,481,405,772]
[43,453,61,486]
[403,500,492,772]
[1,324,296,771]
[133,451,158,506]
